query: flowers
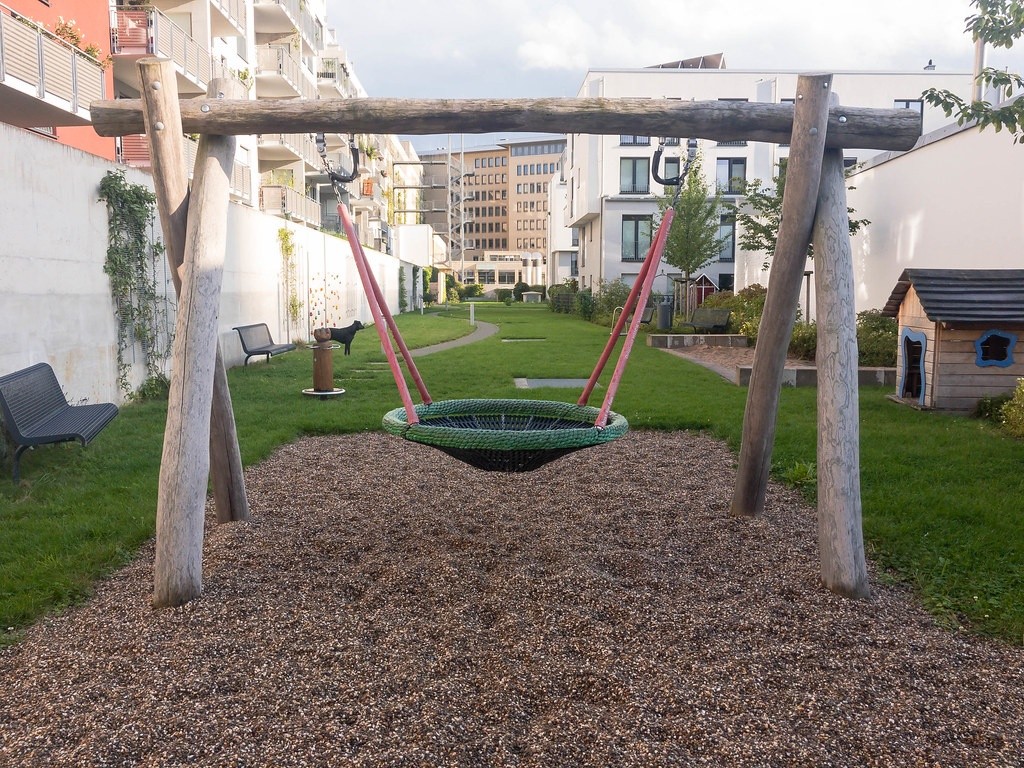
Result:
[14,11,111,71]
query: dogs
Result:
[329,320,364,355]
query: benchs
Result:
[0,362,120,484]
[628,308,654,327]
[232,323,296,366]
[677,308,730,334]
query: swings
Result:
[314,133,698,473]
